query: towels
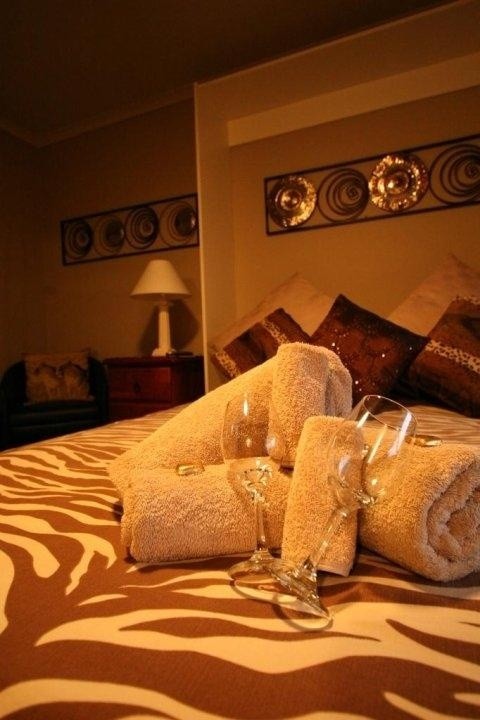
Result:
[107,341,480,581]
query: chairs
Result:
[0,358,106,451]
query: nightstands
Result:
[103,355,203,423]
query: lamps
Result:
[130,258,192,356]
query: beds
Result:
[0,388,480,720]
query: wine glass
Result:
[220,392,296,584]
[255,394,419,620]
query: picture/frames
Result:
[262,132,480,235]
[59,191,199,266]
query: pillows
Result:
[406,295,480,418]
[210,307,311,383]
[311,293,431,418]
[21,346,94,405]
